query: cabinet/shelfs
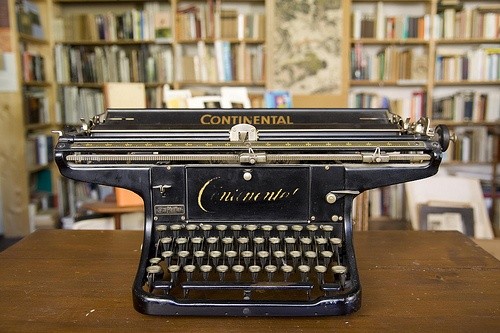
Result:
[0,0,499,240]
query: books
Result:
[15,0,500,230]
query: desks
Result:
[0,230,500,333]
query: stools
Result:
[417,201,476,240]
[85,186,144,230]
